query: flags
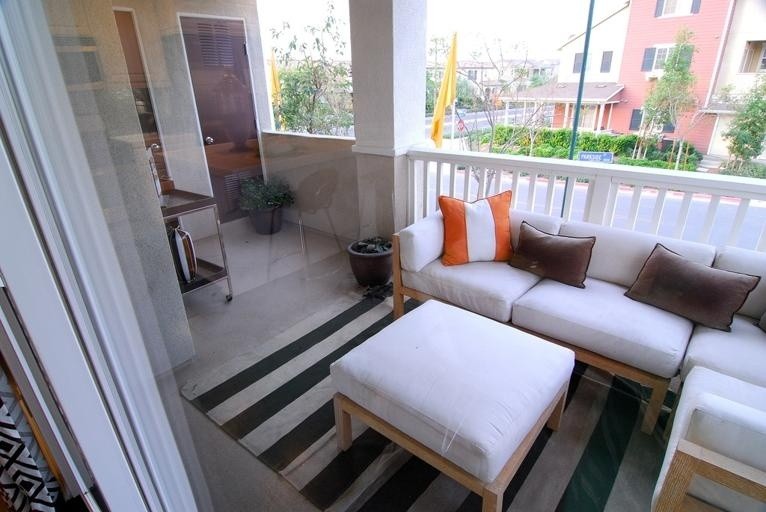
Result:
[431,32,457,147]
[271,47,281,104]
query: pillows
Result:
[433,192,515,268]
[506,220,597,290]
[621,241,762,334]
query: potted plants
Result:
[344,230,396,285]
[233,175,293,232]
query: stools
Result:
[328,296,579,512]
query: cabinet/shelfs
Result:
[160,182,235,303]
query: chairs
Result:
[261,167,347,286]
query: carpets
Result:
[177,280,671,512]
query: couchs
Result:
[389,192,766,508]
